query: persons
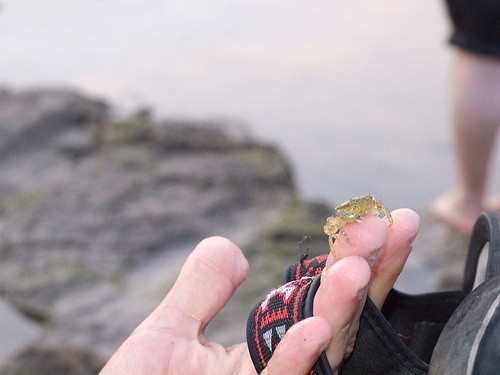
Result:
[429,0,500,239]
[96,208,421,375]
